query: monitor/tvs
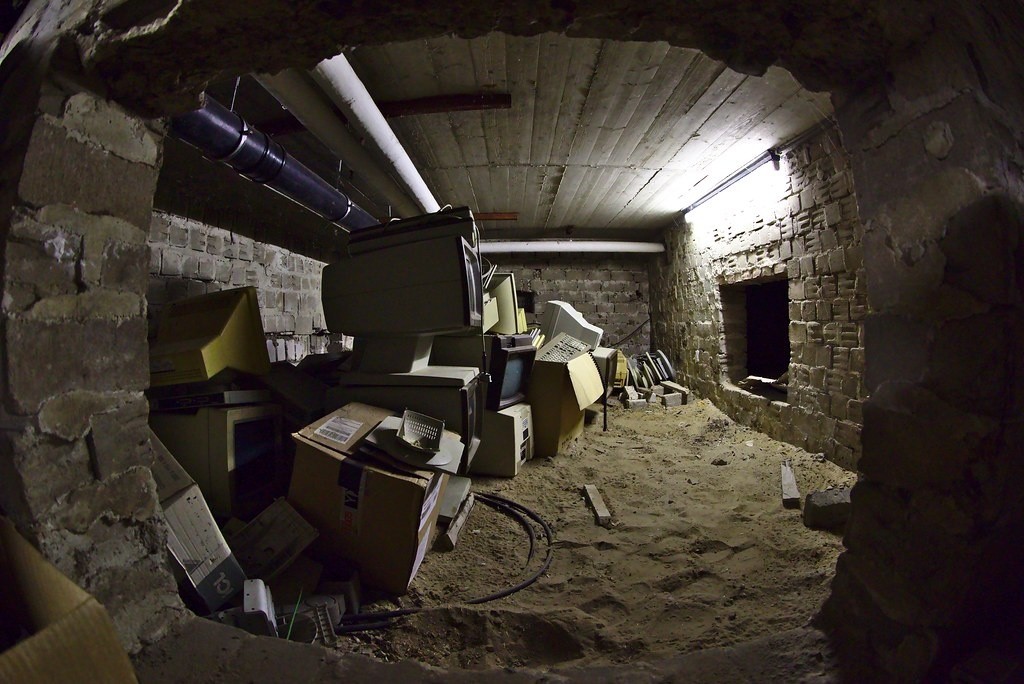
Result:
[146,286,271,398]
[321,234,606,410]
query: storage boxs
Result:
[289,402,450,595]
[226,494,319,584]
[0,515,139,684]
[147,424,248,613]
[526,351,605,456]
[396,408,446,461]
